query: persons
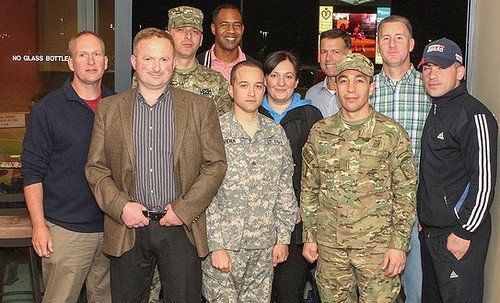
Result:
[83,27,228,303]
[364,14,437,303]
[352,21,368,55]
[300,53,418,303]
[21,29,121,303]
[200,60,299,303]
[414,38,500,303]
[303,28,356,303]
[256,51,328,303]
[196,5,267,82]
[131,5,234,303]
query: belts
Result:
[142,211,166,222]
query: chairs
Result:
[1,191,44,303]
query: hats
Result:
[417,38,463,69]
[334,53,374,78]
[167,6,203,33]
